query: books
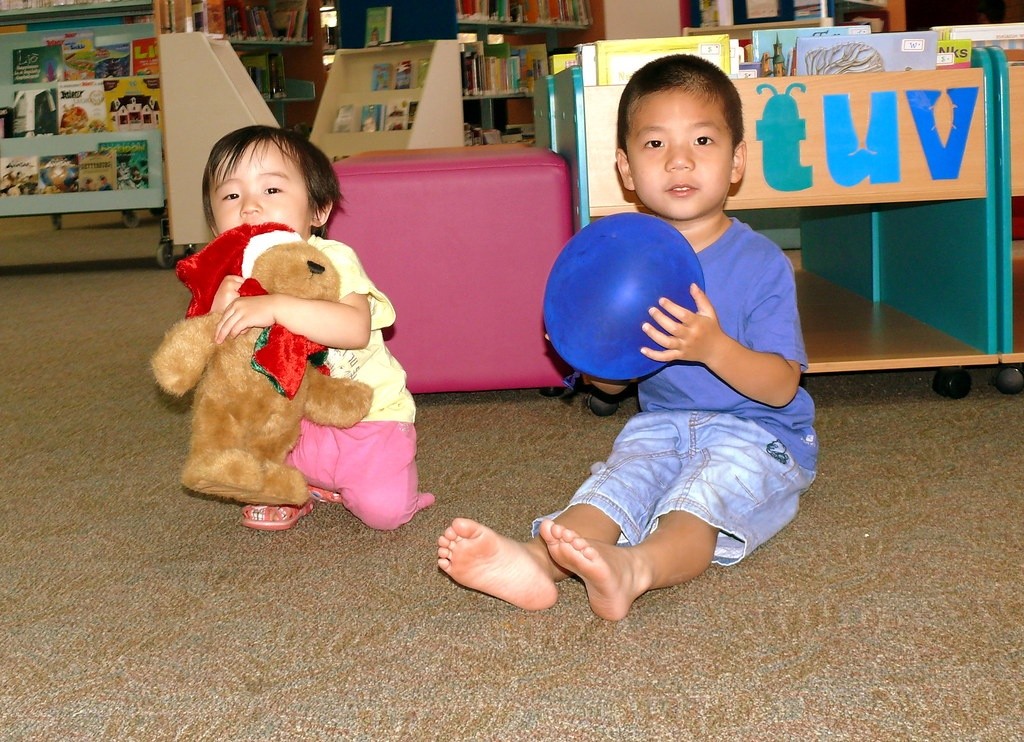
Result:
[364,6,393,49]
[457,0,591,26]
[687,0,891,33]
[184,0,309,100]
[370,58,431,92]
[464,122,536,146]
[332,99,419,132]
[0,0,161,197]
[460,48,544,96]
[550,22,1024,86]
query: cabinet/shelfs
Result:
[1,1,1024,414]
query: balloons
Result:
[543,212,706,381]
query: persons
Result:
[438,54,818,621]
[202,124,435,531]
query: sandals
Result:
[309,486,344,505]
[241,486,314,531]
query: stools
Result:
[320,148,571,398]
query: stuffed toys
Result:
[148,222,373,506]
